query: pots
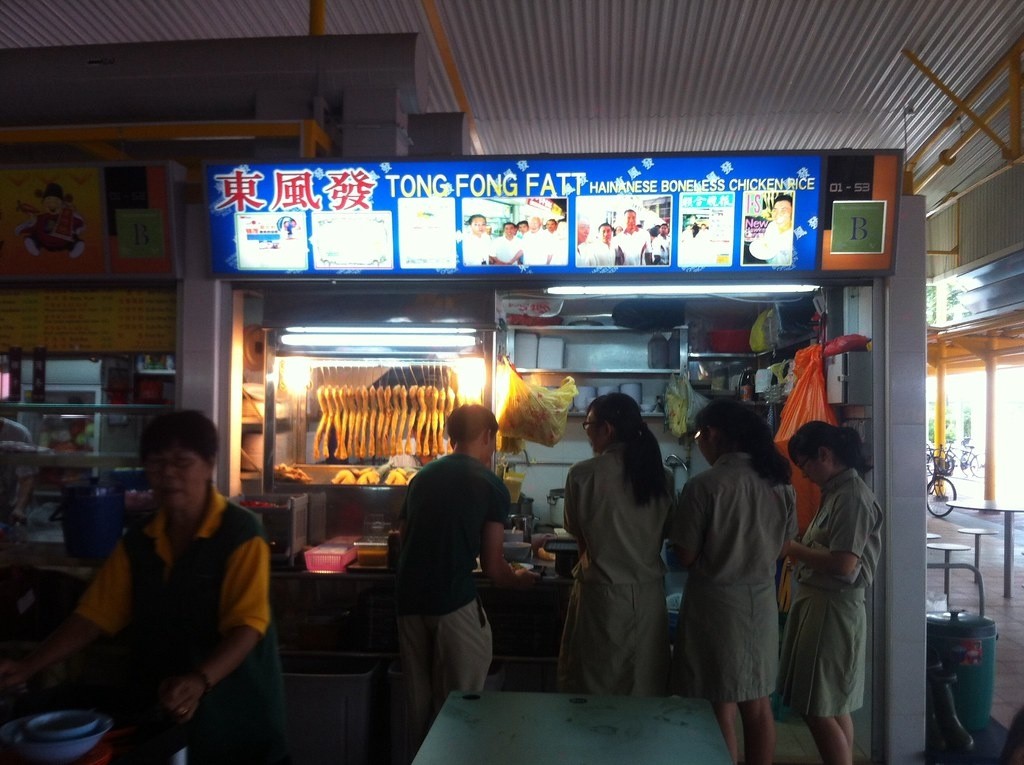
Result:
[545,489,567,527]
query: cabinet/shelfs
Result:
[505,323,687,420]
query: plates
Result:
[507,563,533,571]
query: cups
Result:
[515,515,532,544]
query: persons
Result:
[396,408,525,765]
[0,408,292,764]
[679,214,709,266]
[743,195,794,265]
[660,399,799,764]
[465,214,567,266]
[577,208,671,267]
[781,420,883,765]
[557,394,680,698]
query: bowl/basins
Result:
[542,383,655,413]
[2,715,113,761]
[28,709,98,740]
[504,542,532,562]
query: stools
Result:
[927,543,971,607]
[958,528,999,583]
[926,533,941,539]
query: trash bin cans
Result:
[927,607,998,731]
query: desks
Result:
[946,500,1024,599]
[412,691,734,765]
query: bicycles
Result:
[926,436,985,519]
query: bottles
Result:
[739,367,754,401]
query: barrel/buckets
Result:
[928,609,997,731]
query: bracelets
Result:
[191,666,213,694]
